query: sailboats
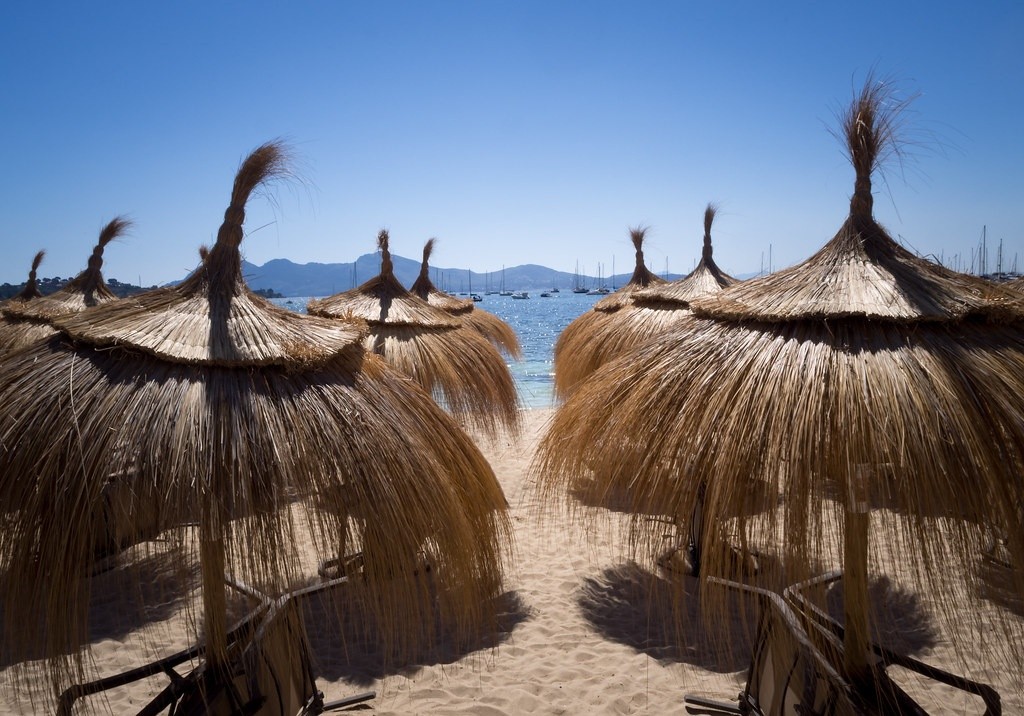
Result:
[435,264,512,301]
[969,225,1022,281]
[573,254,618,295]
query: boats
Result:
[551,288,560,292]
[286,301,293,304]
[511,292,529,299]
[541,292,552,297]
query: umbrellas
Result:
[542,91,1023,678]
[1,143,517,716]
[553,202,753,471]
[0,250,46,310]
[0,208,132,352]
[407,238,520,364]
[552,227,670,360]
[307,229,523,441]
